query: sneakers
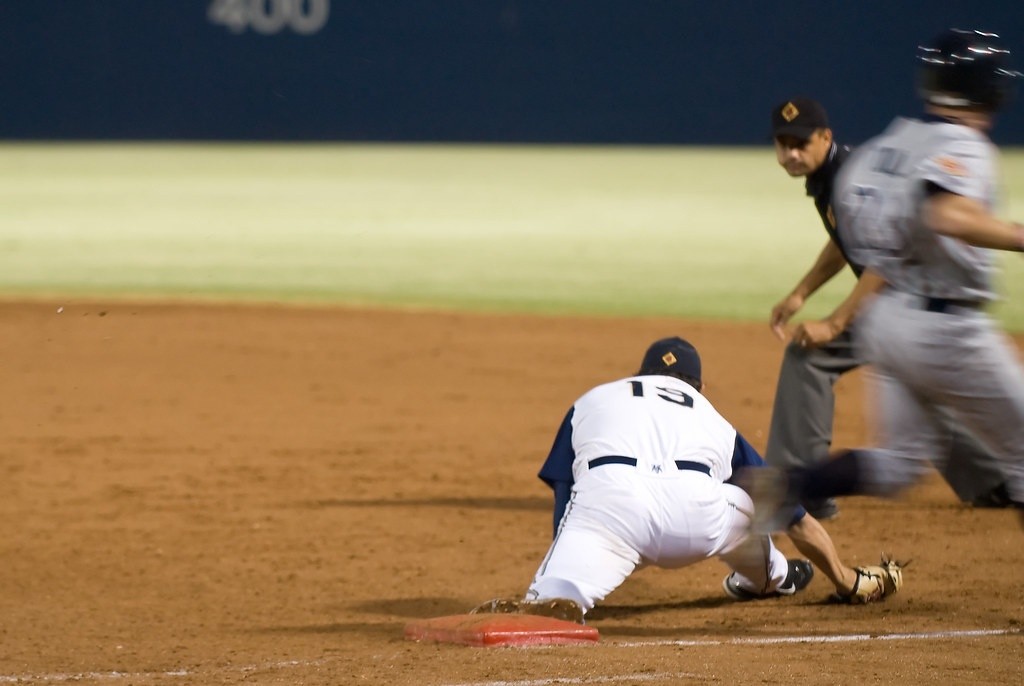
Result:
[723,558,814,599]
[469,598,583,627]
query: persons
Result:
[470,336,903,627]
[737,25,1024,525]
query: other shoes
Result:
[974,484,1013,508]
[809,501,839,518]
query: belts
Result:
[588,455,711,478]
[925,297,983,313]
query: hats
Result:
[771,98,830,138]
[640,336,701,381]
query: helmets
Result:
[914,26,1024,110]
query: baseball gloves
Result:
[843,559,903,605]
